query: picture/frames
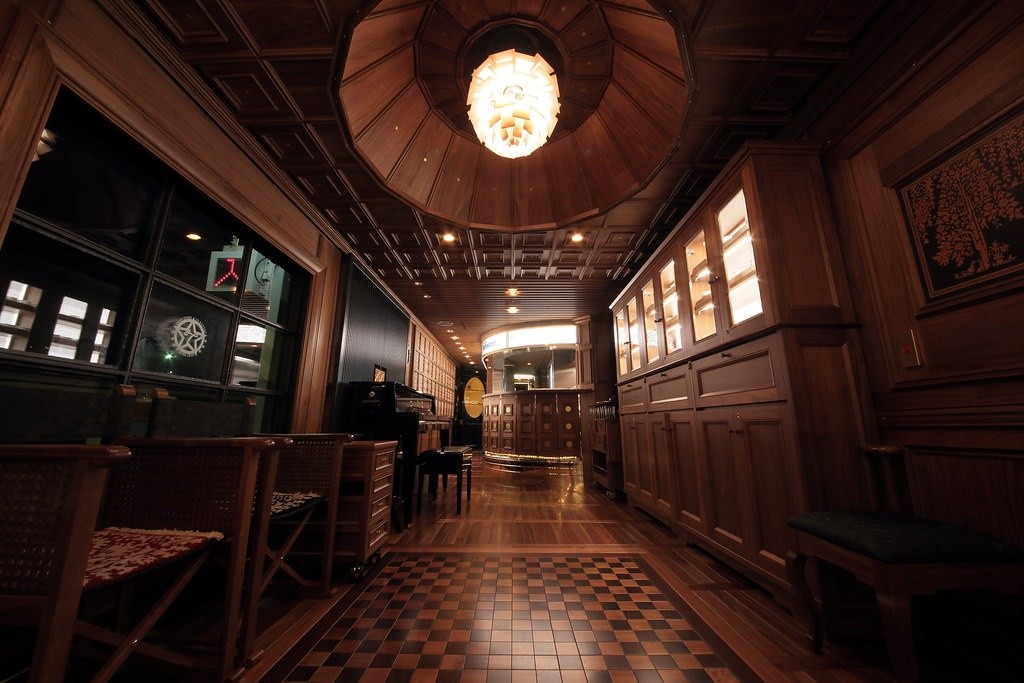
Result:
[884,96,1023,321]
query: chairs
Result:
[0,385,355,683]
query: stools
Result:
[785,509,1024,683]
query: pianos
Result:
[342,379,454,530]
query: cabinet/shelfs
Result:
[587,395,624,498]
[329,440,399,578]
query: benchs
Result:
[417,446,473,515]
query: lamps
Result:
[466,48,560,159]
